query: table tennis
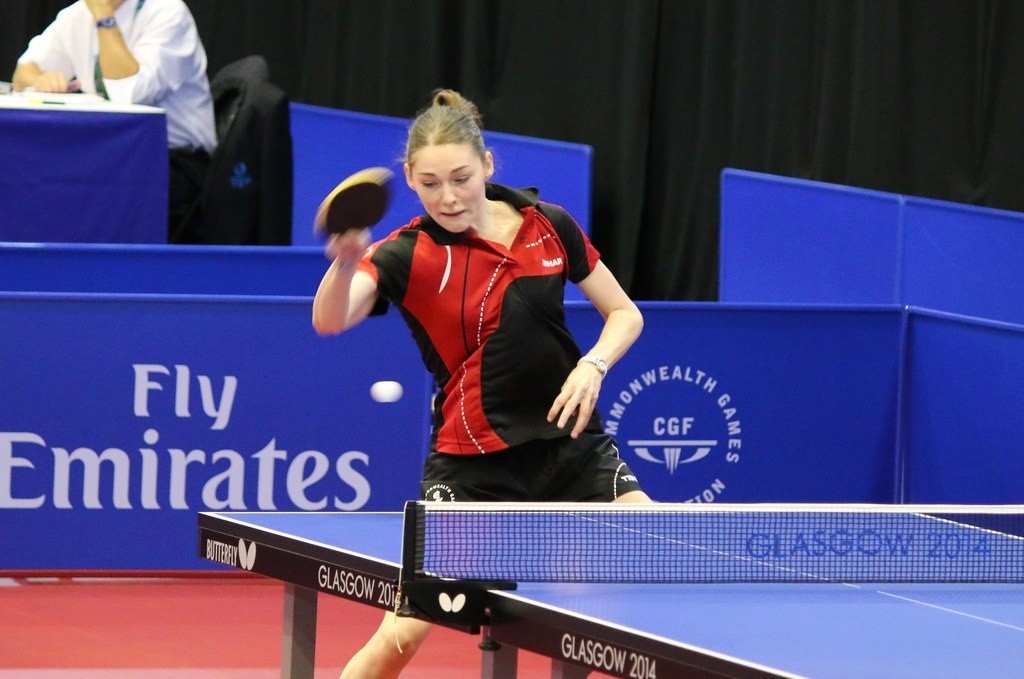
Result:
[369,380,403,404]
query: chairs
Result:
[167,52,270,243]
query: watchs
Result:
[97,16,115,28]
[577,357,608,379]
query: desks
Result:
[198,498,1024,679]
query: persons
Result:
[310,91,656,679]
[9,0,220,243]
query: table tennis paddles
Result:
[327,181,387,254]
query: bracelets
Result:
[332,259,357,273]
